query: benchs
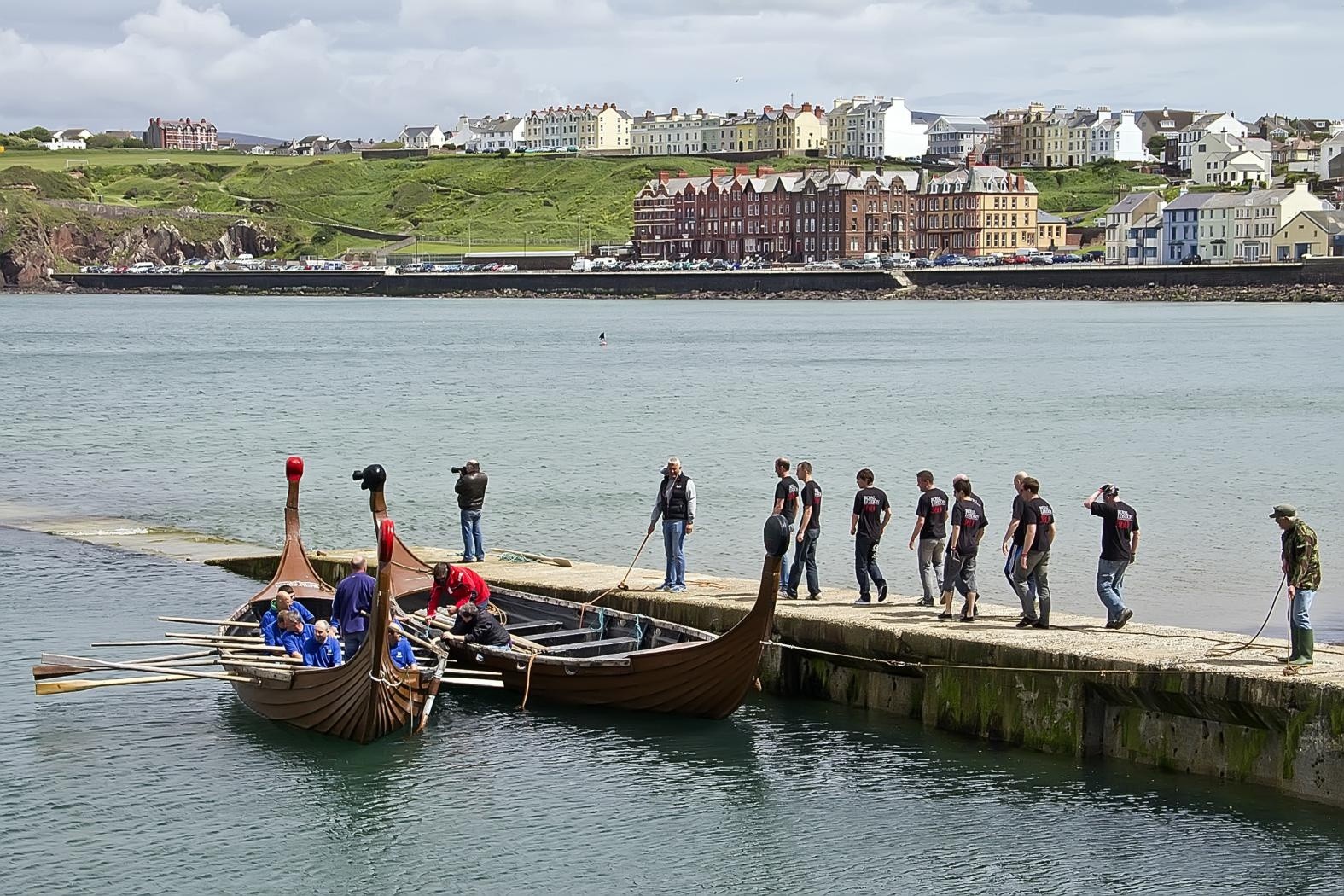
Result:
[505,619,564,632]
[520,627,593,644]
[547,636,637,656]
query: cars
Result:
[968,252,1016,267]
[881,252,937,269]
[933,254,972,267]
[823,154,838,159]
[843,258,884,271]
[396,263,518,272]
[620,261,699,270]
[805,260,840,270]
[179,254,281,270]
[692,260,772,271]
[283,262,364,270]
[1014,248,1053,266]
[875,155,893,162]
[79,262,186,274]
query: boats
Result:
[352,464,790,727]
[219,457,427,741]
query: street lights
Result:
[1145,211,1149,265]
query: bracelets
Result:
[688,522,693,524]
[1021,554,1026,556]
[953,547,956,548]
[1131,552,1135,555]
[1098,489,1102,492]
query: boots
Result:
[1278,627,1299,663]
[1289,628,1314,665]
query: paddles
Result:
[414,604,552,653]
[362,610,455,658]
[407,653,505,688]
[32,616,345,696]
[489,548,573,567]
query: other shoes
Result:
[961,592,979,613]
[937,610,952,621]
[1114,608,1133,630]
[1019,611,1025,617]
[1016,617,1036,628]
[779,586,789,592]
[964,603,978,616]
[457,558,474,563]
[960,613,975,623]
[476,559,483,562]
[1105,621,1116,629]
[1031,617,1049,629]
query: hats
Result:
[389,622,401,631]
[1102,485,1117,498]
[1269,504,1296,519]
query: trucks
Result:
[570,258,624,271]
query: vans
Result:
[908,157,922,164]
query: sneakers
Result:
[654,583,670,591]
[805,592,822,599]
[669,585,686,593]
[855,597,870,604]
[917,597,934,606]
[777,591,795,599]
[940,590,947,604]
[878,584,888,602]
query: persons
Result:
[455,460,488,562]
[1013,478,1056,630]
[1083,484,1139,629]
[778,461,822,600]
[1002,472,1035,617]
[425,562,491,635]
[953,473,984,615]
[771,457,799,591]
[442,602,512,648]
[647,456,697,593]
[1269,504,1321,666]
[262,555,418,670]
[851,469,892,604]
[938,479,988,623]
[909,470,949,607]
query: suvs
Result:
[1020,162,1036,167]
[1170,179,1199,187]
[1053,250,1105,263]
[958,160,966,166]
[938,158,956,165]
[1179,253,1201,265]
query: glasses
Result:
[1020,488,1029,492]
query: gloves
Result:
[1100,484,1111,491]
[1129,553,1136,564]
